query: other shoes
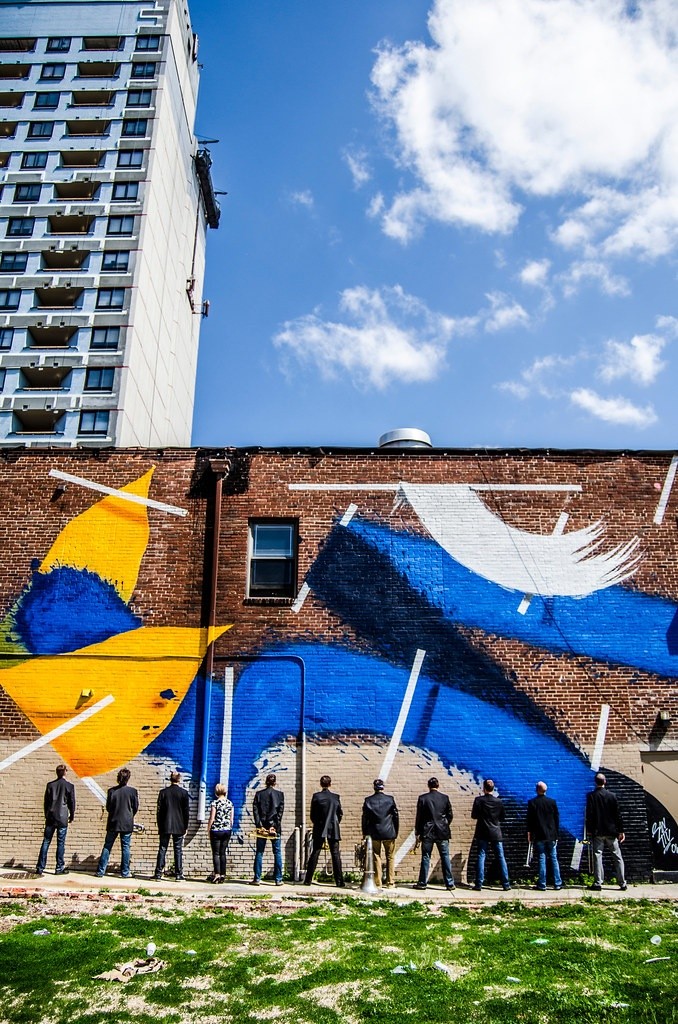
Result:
[93,872,102,877]
[587,885,601,891]
[532,885,546,891]
[249,881,260,885]
[447,886,456,890]
[472,884,481,891]
[122,872,136,878]
[176,875,186,880]
[386,883,395,888]
[621,886,628,891]
[303,880,311,885]
[554,885,562,890]
[35,869,43,874]
[413,884,426,890]
[150,875,161,879]
[56,869,69,874]
[277,881,284,886]
[504,886,511,890]
[337,883,346,888]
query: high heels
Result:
[220,877,225,883]
[211,877,220,884]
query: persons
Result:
[585,773,627,891]
[149,772,190,882]
[470,780,511,890]
[362,779,400,888]
[96,769,139,877]
[35,765,75,875]
[303,776,345,887]
[413,778,456,891]
[208,783,234,884]
[526,781,564,890]
[248,775,284,886]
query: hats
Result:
[374,779,384,790]
